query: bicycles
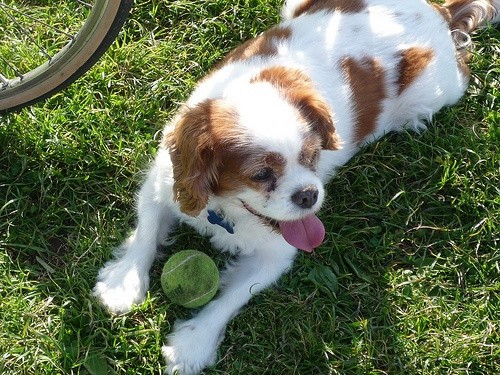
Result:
[1,0,133,117]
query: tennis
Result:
[161,250,220,307]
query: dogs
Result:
[93,0,500,375]
